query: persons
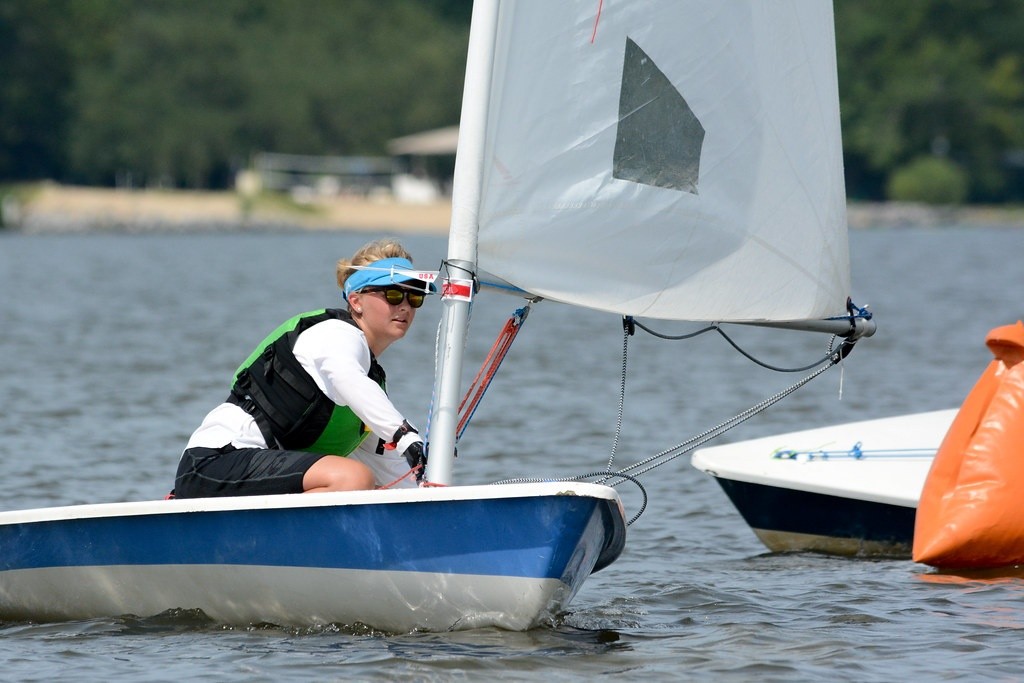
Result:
[167,236,438,501]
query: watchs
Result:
[383,417,419,451]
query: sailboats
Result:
[0,0,887,636]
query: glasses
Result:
[356,285,424,308]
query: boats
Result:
[690,407,968,560]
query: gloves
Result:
[403,441,427,486]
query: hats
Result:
[343,258,437,304]
